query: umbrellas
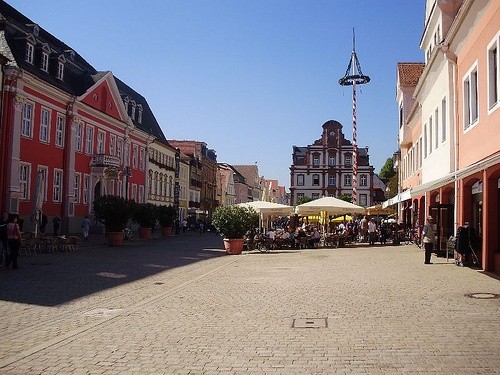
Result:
[234,196,396,239]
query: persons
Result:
[422,216,435,264]
[39,213,48,234]
[266,216,397,249]
[0,210,22,271]
[53,215,60,237]
[170,218,208,235]
[81,216,90,240]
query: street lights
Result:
[338,27,370,205]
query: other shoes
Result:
[12,268,20,270]
[425,263,433,264]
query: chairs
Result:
[18,231,82,257]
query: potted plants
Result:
[92,196,179,247]
[211,204,260,255]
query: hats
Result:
[427,216,434,220]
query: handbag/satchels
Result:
[423,237,431,243]
